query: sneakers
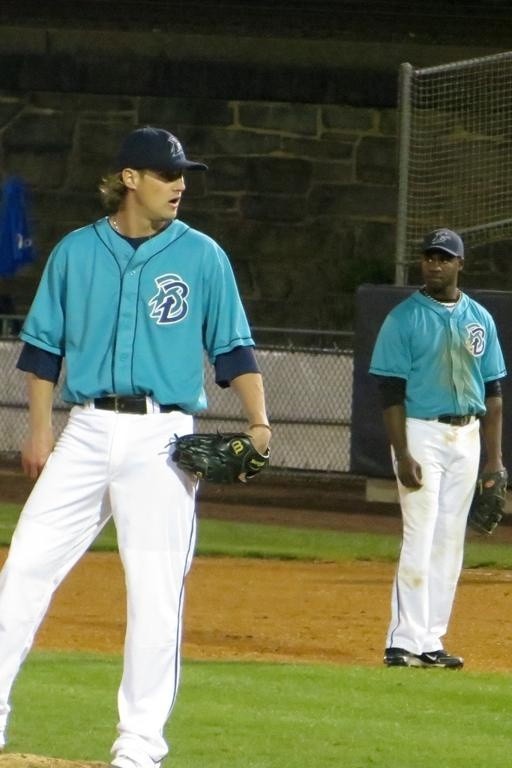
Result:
[382,648,410,666]
[411,649,465,668]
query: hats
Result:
[418,229,464,258]
[114,128,209,176]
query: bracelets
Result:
[249,424,273,434]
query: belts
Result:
[437,413,481,427]
[93,395,182,414]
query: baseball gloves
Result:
[466,469,509,536]
[171,431,269,484]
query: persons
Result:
[0,126,274,768]
[368,227,510,671]
